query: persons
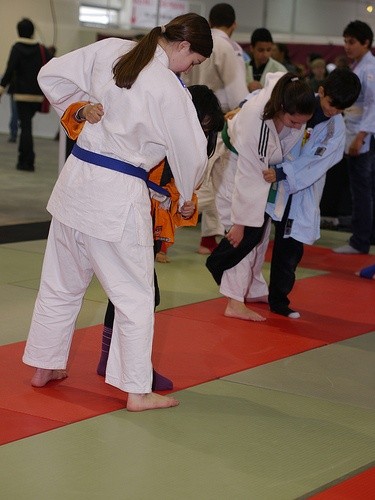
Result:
[59,85,225,390]
[332,20,375,254]
[205,67,362,318]
[22,12,214,411]
[244,27,287,93]
[269,42,352,84]
[180,3,250,255]
[206,71,317,322]
[0,19,51,172]
